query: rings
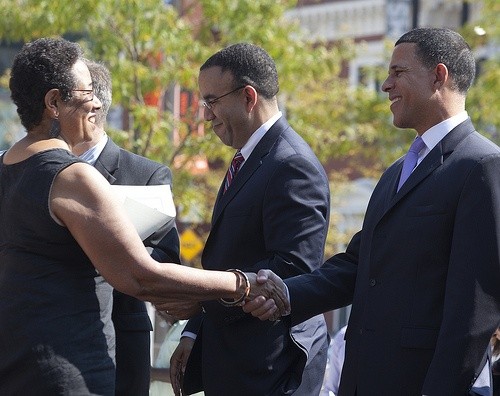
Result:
[166,309,169,315]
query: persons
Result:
[0,38,289,396]
[70,59,183,396]
[154,42,329,396]
[243,27,499,396]
[320,320,500,396]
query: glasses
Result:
[62,89,95,99]
[202,85,245,110]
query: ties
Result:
[223,151,244,195]
[397,136,425,194]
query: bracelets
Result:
[215,268,250,306]
